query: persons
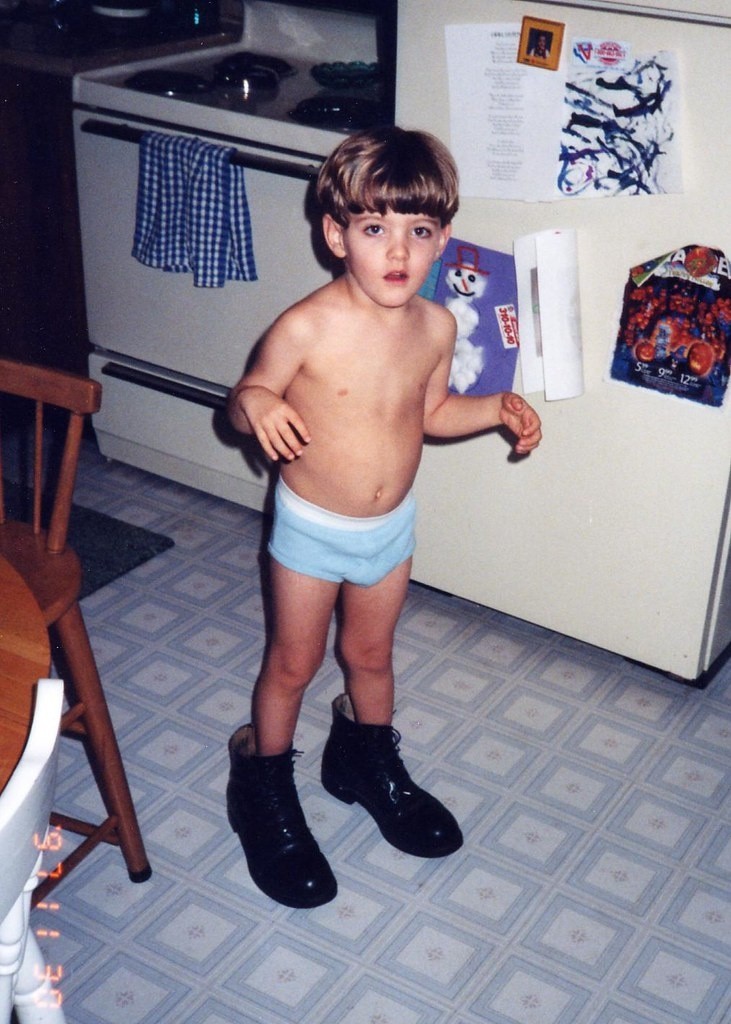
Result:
[225,126,542,909]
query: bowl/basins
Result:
[311,62,380,89]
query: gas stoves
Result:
[73,40,394,176]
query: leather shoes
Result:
[226,723,338,909]
[322,693,464,857]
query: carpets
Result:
[0,475,173,601]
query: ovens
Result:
[73,114,377,514]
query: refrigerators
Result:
[393,0,731,680]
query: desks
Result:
[0,555,52,794]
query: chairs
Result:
[0,678,64,1024]
[0,360,154,913]
